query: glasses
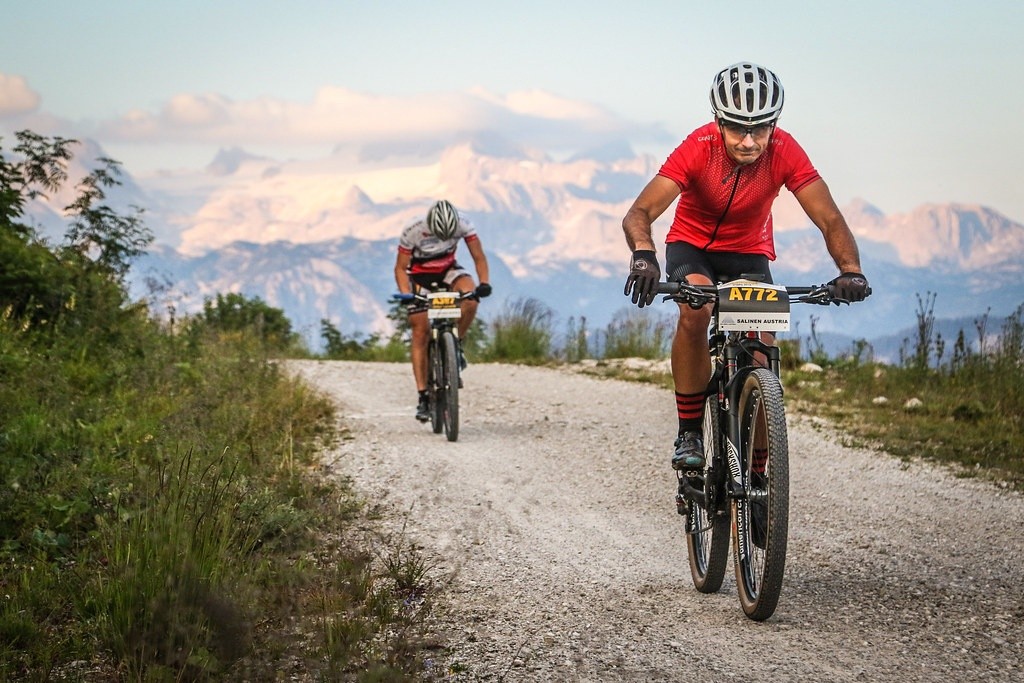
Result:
[718,118,776,141]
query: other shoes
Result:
[416,396,433,420]
[461,349,467,370]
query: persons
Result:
[394,200,492,420]
[622,62,868,551]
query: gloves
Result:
[475,283,492,298]
[401,298,421,307]
[826,272,871,307]
[624,249,661,308]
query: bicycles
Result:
[635,281,872,621]
[395,291,482,443]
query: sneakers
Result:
[671,431,707,468]
[751,478,769,550]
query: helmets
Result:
[427,199,461,242]
[710,62,785,126]
